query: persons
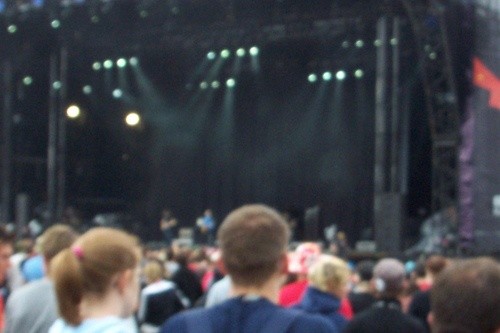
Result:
[1,202,499,332]
